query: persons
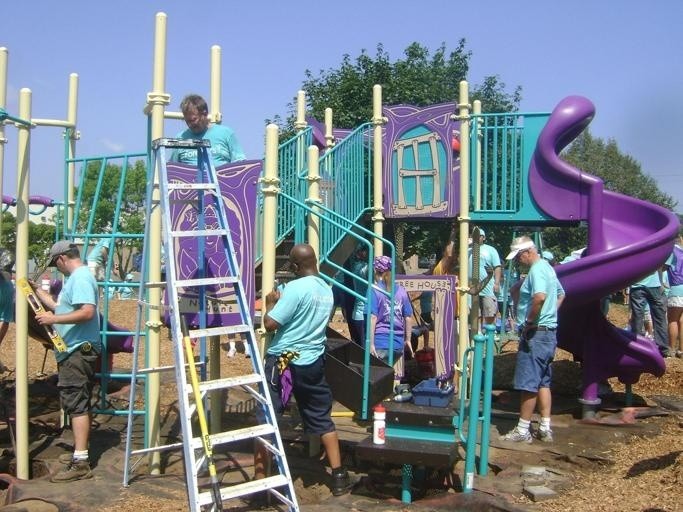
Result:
[27,241,102,482]
[101,278,116,301]
[0,272,14,347]
[340,228,589,389]
[170,94,245,166]
[241,244,362,497]
[118,273,134,300]
[275,276,287,292]
[498,235,565,444]
[610,235,683,358]
[227,332,250,359]
[86,232,123,297]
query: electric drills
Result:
[382,389,413,403]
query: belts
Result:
[529,327,547,330]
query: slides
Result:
[528,97,681,379]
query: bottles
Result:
[372,402,387,445]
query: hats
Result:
[479,229,485,236]
[373,256,391,272]
[127,274,134,279]
[506,241,534,260]
[47,240,77,267]
[538,251,553,260]
[559,256,574,264]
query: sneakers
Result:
[227,348,237,358]
[51,453,93,483]
[332,470,353,496]
[245,349,250,357]
[499,427,552,443]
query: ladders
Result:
[122,139,297,512]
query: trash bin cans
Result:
[132,254,142,272]
